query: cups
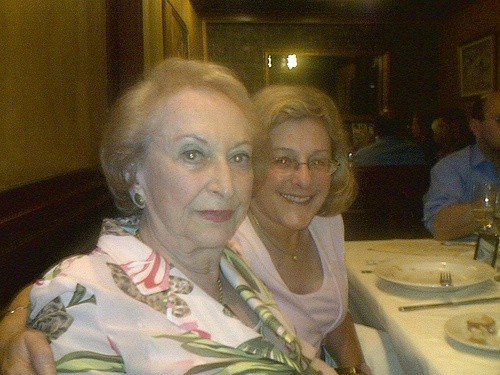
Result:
[471,183,500,236]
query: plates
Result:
[374,261,495,292]
[444,310,500,351]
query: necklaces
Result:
[249,207,302,261]
[136,230,224,303]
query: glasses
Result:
[271,156,341,178]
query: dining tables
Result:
[344,238,500,375]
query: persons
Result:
[422,92,500,241]
[27,57,340,375]
[413,113,477,152]
[0,85,374,375]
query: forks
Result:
[439,271,452,286]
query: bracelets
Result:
[4,305,27,315]
[334,366,363,375]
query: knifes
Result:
[398,297,500,311]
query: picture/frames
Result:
[162,0,189,60]
[457,34,495,97]
[262,46,390,122]
